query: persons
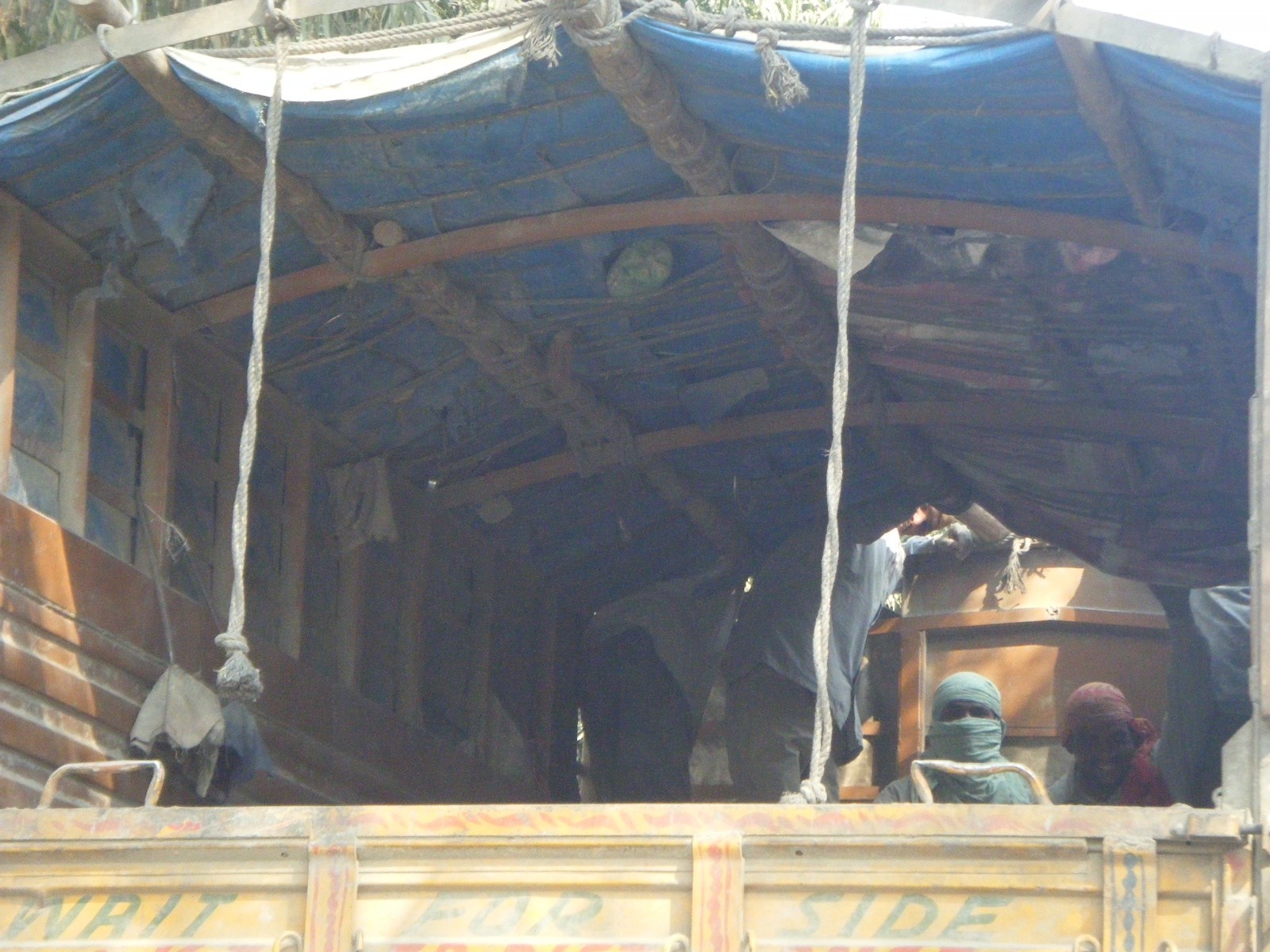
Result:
[1045,682,1171,805]
[873,671,1039,804]
[724,445,973,802]
